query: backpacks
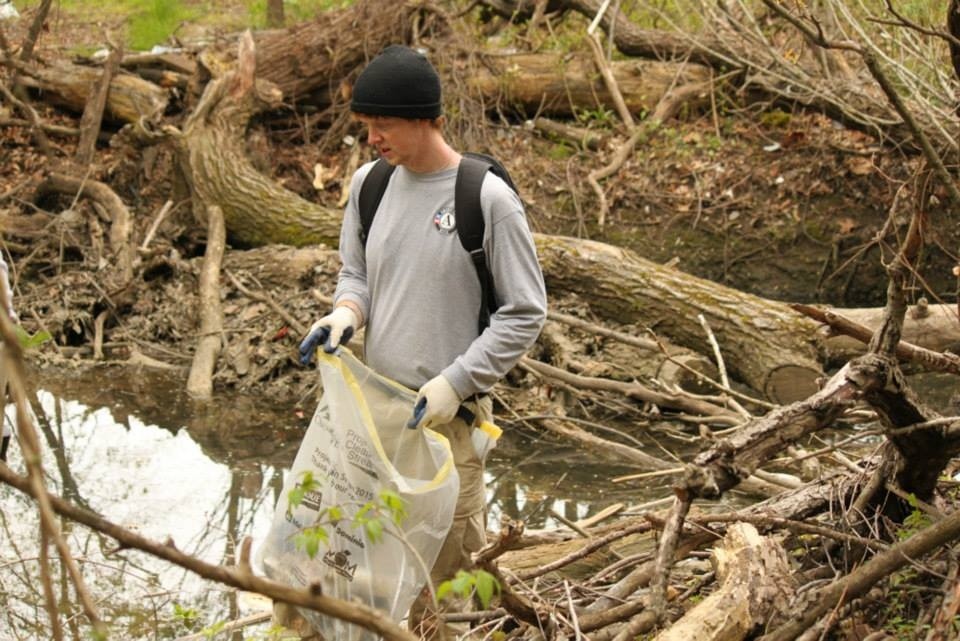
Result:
[359,152,519,337]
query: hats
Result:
[350,46,441,117]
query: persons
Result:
[265,43,548,641]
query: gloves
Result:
[300,306,358,369]
[407,374,461,428]
